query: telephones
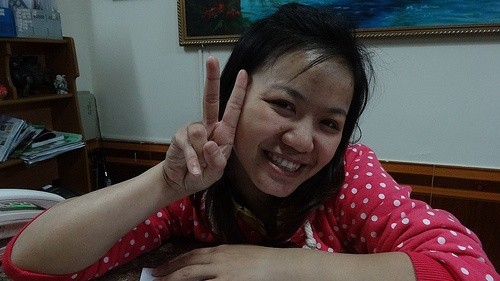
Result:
[0,189,66,240]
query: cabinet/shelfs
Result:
[0,35,91,194]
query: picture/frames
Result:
[177,0,500,46]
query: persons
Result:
[2,3,500,281]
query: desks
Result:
[0,230,217,281]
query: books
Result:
[0,116,85,164]
